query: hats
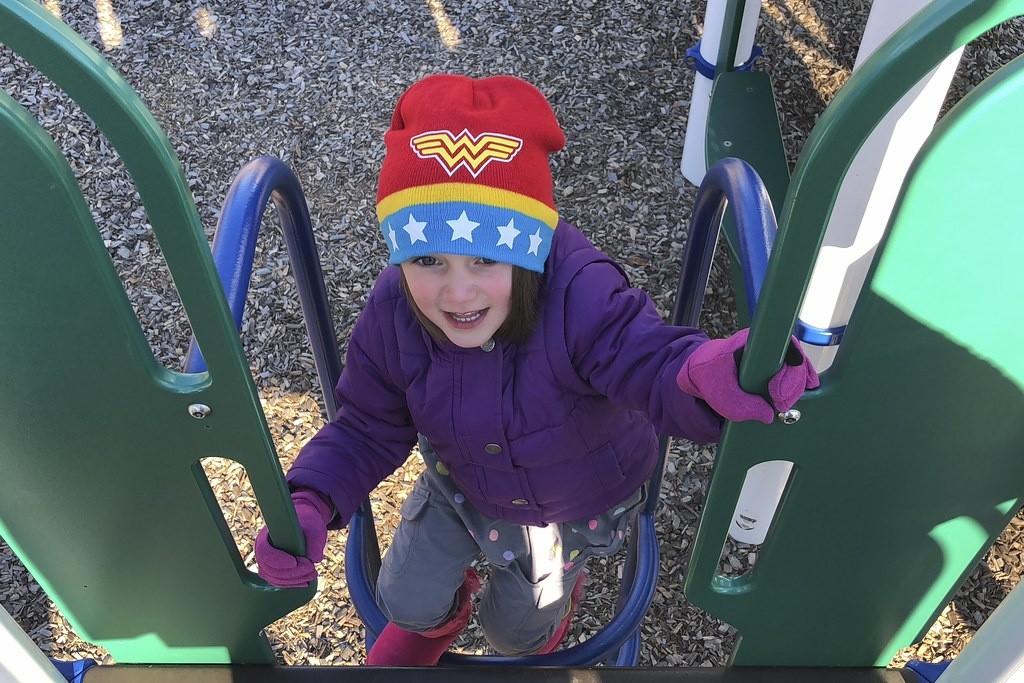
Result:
[376,74,564,272]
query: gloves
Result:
[677,328,820,424]
[255,491,337,588]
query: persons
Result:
[255,74,820,666]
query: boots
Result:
[367,568,479,666]
[533,571,584,653]
